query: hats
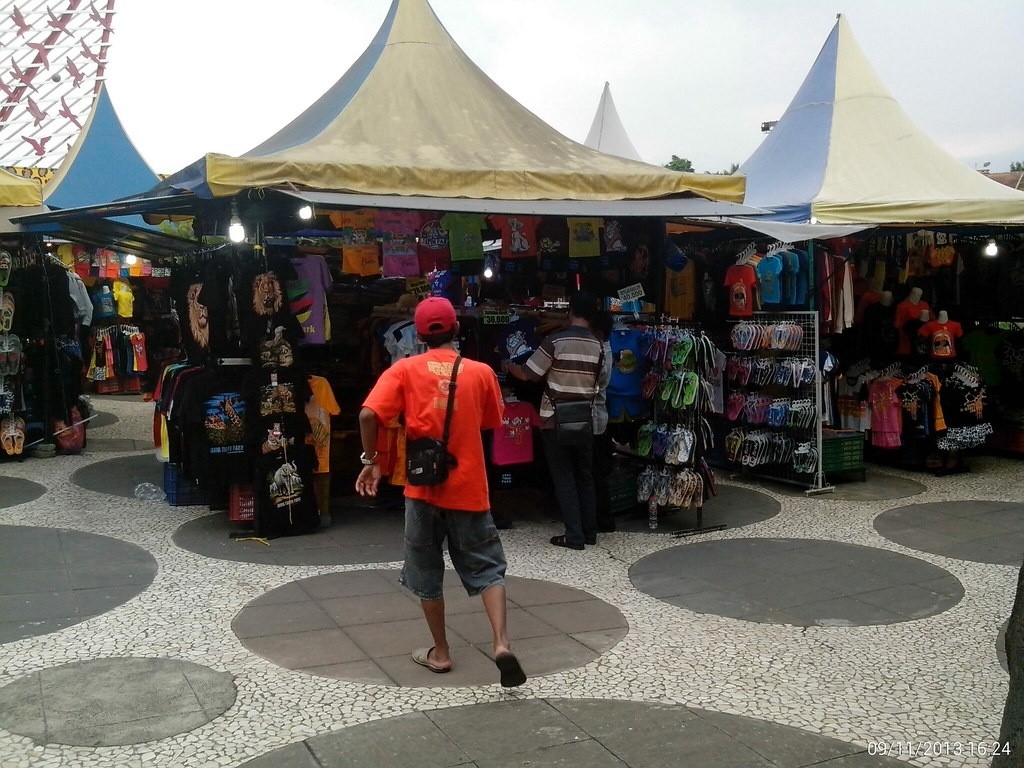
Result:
[412,296,457,336]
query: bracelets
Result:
[359,450,377,464]
[504,361,516,373]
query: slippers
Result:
[411,643,452,674]
[725,324,820,474]
[495,651,527,688]
[632,328,717,514]
[0,249,26,456]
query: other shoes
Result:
[549,534,596,551]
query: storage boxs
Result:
[775,427,868,487]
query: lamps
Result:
[228,195,245,243]
[986,233,997,256]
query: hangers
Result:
[610,312,813,478]
[506,308,520,323]
[735,240,793,265]
[813,242,851,258]
[505,393,520,403]
[11,250,37,272]
[90,323,139,338]
[847,357,981,386]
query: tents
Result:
[42,80,177,242]
[583,79,643,164]
[727,13,1024,325]
[0,166,63,234]
[142,0,747,260]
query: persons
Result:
[354,297,526,687]
[902,309,934,369]
[916,310,963,379]
[113,285,135,318]
[854,278,884,323]
[862,291,900,366]
[492,378,543,528]
[92,285,117,318]
[497,291,606,551]
[893,287,935,375]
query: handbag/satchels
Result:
[405,435,458,487]
[553,400,594,454]
